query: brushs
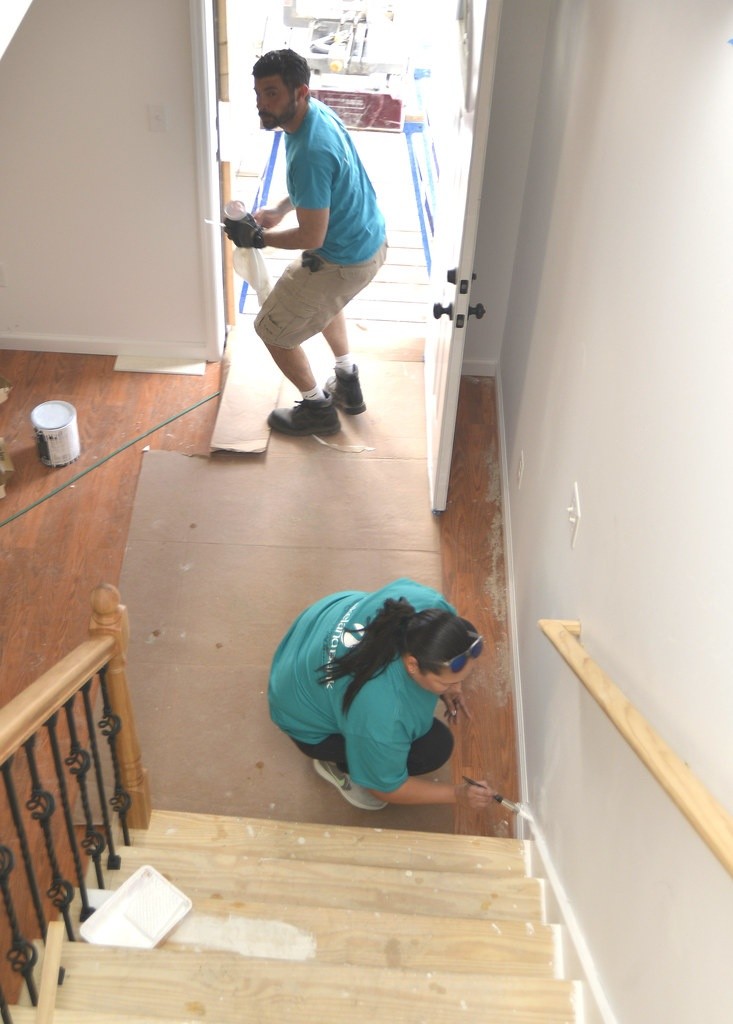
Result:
[203,218,226,227]
[461,776,532,821]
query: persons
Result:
[224,47,387,437]
[267,576,494,811]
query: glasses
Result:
[419,631,483,673]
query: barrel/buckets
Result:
[30,401,80,467]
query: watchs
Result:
[253,227,265,248]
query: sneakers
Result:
[313,756,388,811]
[269,389,340,436]
[323,364,366,415]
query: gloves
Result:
[224,212,259,249]
